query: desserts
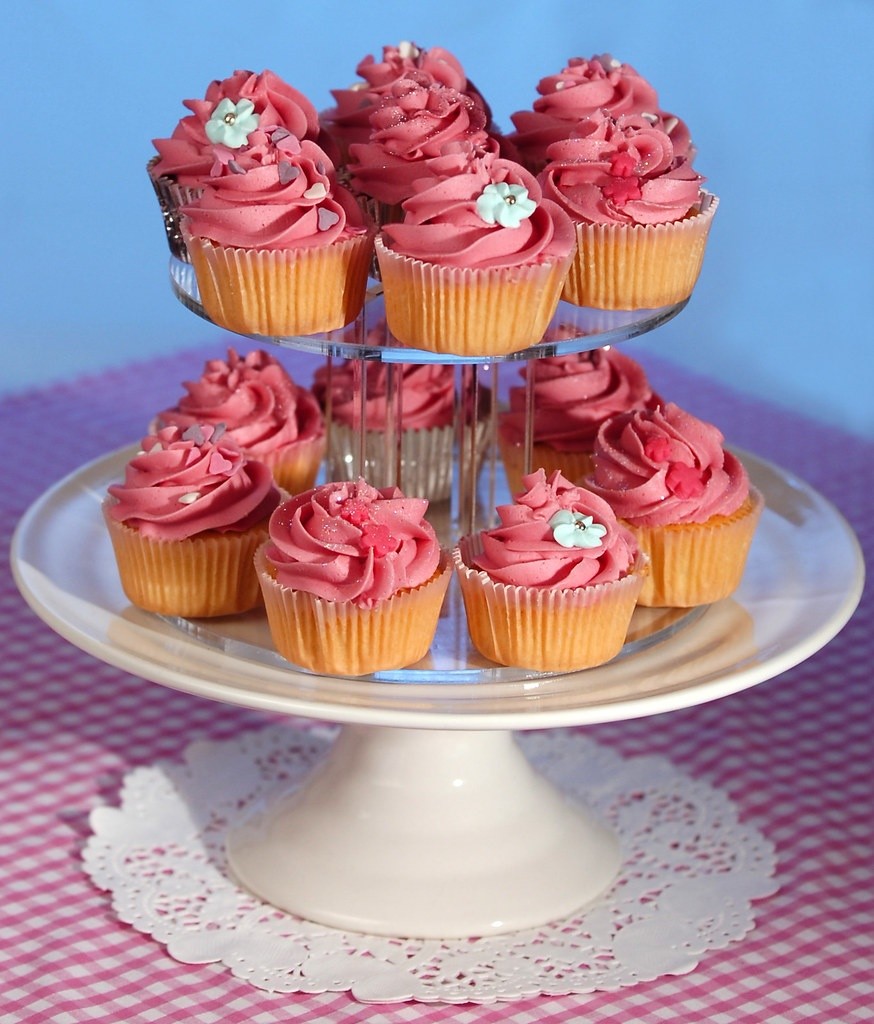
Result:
[143,42,719,357]
[102,315,764,677]
